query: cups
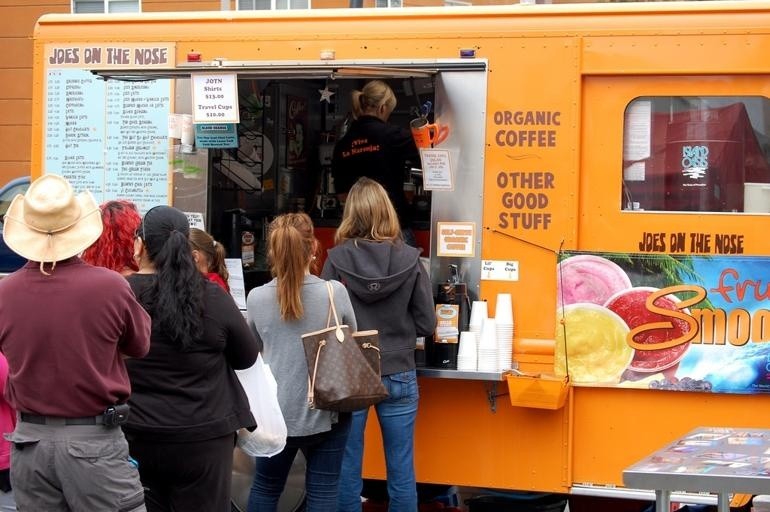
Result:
[403,182,416,202]
[411,118,439,150]
[557,255,694,387]
[456,293,514,372]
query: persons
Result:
[328,79,422,243]
[317,175,440,511]
[117,202,263,512]
[1,353,20,511]
[187,224,233,292]
[0,175,158,512]
[289,116,305,160]
[77,198,147,280]
[243,211,364,511]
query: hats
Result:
[2,174,103,263]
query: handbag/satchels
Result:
[104,404,130,429]
[301,281,389,412]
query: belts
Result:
[21,413,103,425]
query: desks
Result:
[623,426,770,512]
